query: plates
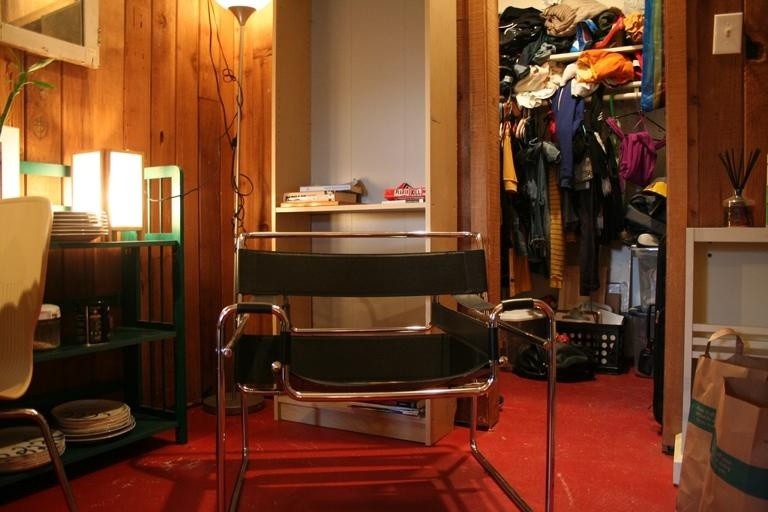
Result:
[50,210,108,243]
[49,399,137,442]
[0,426,65,472]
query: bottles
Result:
[721,189,756,227]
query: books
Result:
[384,186,425,200]
[382,199,425,205]
[345,398,425,420]
[278,177,366,210]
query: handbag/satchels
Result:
[673,321,767,512]
[512,336,600,383]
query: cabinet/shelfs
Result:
[267,0,461,448]
[0,160,186,490]
[667,225,768,487]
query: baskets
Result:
[522,305,629,373]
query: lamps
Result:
[202,0,272,416]
[69,147,147,239]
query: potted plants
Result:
[718,147,762,227]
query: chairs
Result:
[2,197,81,512]
[212,232,562,510]
